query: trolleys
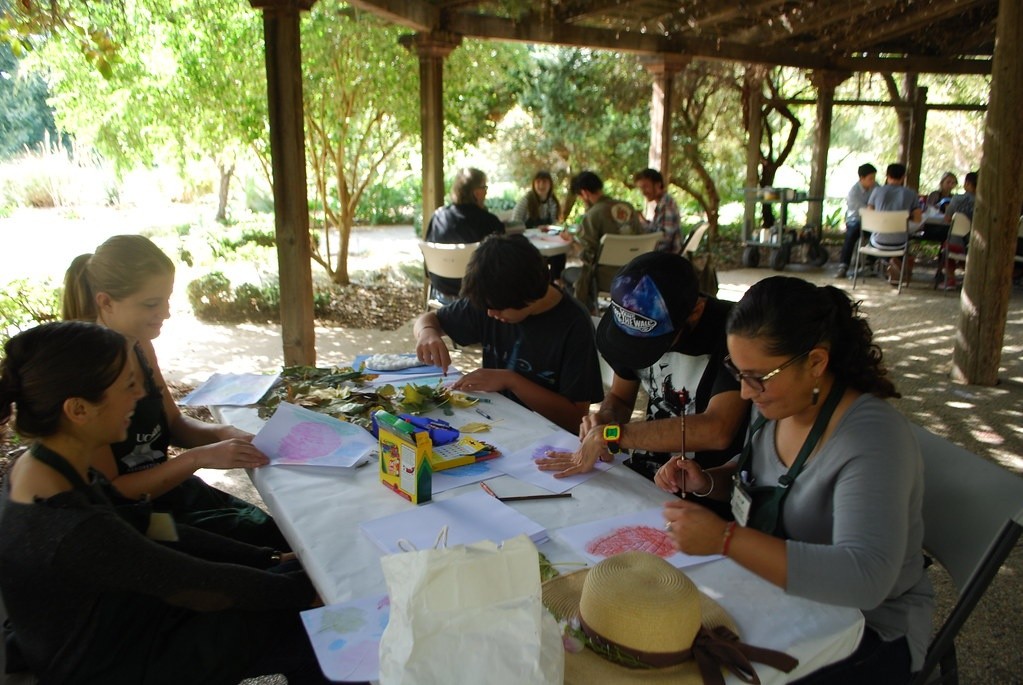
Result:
[736,185,829,272]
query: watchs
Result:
[603,421,625,454]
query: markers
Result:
[466,396,490,403]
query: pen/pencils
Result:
[472,407,490,419]
[480,482,497,498]
[681,409,686,499]
[499,494,572,501]
[474,441,498,457]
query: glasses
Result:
[477,185,489,191]
[723,350,813,393]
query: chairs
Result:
[586,230,664,318]
[677,220,711,264]
[910,421,1023,685]
[934,212,1023,295]
[852,208,912,293]
[416,238,479,348]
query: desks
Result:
[914,212,951,241]
[201,350,868,685]
[518,225,577,281]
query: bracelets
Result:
[271,550,281,566]
[721,521,737,556]
[418,326,441,337]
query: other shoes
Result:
[835,268,846,279]
[851,264,874,277]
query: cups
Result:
[759,227,772,244]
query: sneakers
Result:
[936,278,957,291]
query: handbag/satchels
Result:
[378,524,565,684]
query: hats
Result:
[594,249,700,370]
[541,550,800,684]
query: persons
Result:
[938,172,978,289]
[535,250,754,522]
[654,276,935,685]
[865,163,922,276]
[62,234,290,552]
[634,168,684,254]
[561,171,644,315]
[413,232,605,436]
[423,167,505,306]
[922,171,958,285]
[511,169,567,283]
[833,162,882,278]
[0,320,370,685]
